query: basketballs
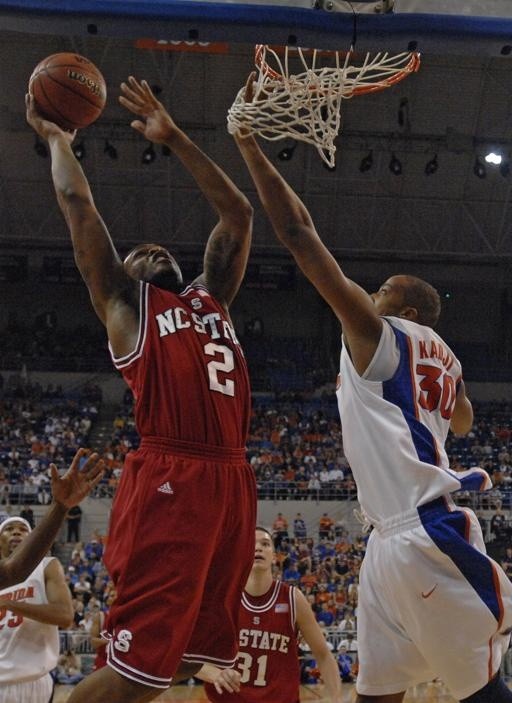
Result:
[25,50,110,131]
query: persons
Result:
[337,646,355,682]
[64,504,83,544]
[227,68,509,701]
[322,632,336,652]
[0,372,22,509]
[298,634,310,651]
[276,542,361,634]
[297,652,323,684]
[55,642,84,686]
[447,398,509,543]
[26,386,112,498]
[22,70,259,701]
[336,634,359,653]
[319,512,335,539]
[192,525,341,703]
[55,532,107,652]
[20,502,35,531]
[292,511,309,540]
[261,397,340,495]
[273,513,289,542]
[0,516,74,703]
